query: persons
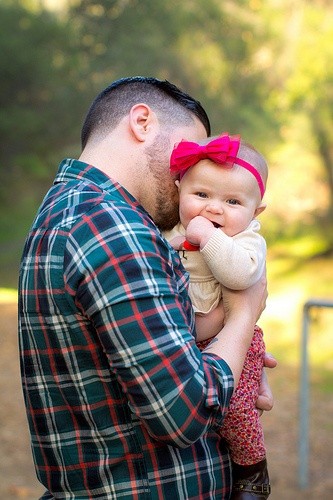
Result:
[18,75,277,500]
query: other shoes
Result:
[232,472,273,500]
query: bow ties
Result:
[169,135,241,176]
